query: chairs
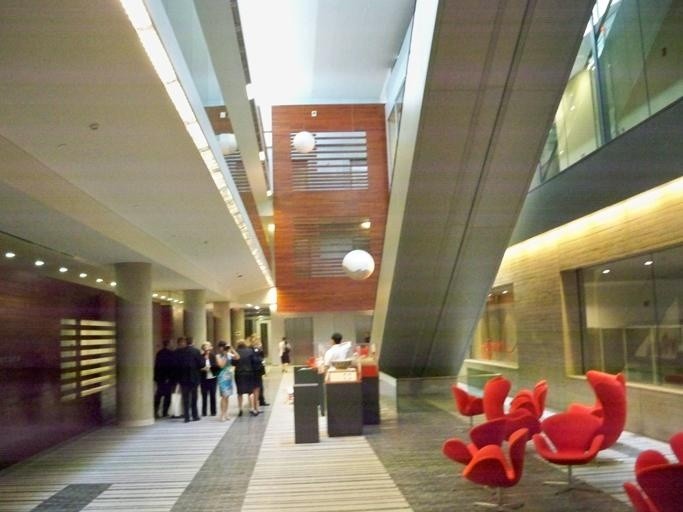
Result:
[440,367,683,512]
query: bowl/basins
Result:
[331,359,351,368]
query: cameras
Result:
[224,345,230,351]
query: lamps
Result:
[292,104,316,156]
[340,104,375,281]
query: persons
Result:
[322,333,352,380]
[153,334,270,423]
[279,337,292,373]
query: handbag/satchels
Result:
[249,347,265,378]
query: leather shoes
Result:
[248,408,265,415]
[152,413,168,420]
[220,415,231,422]
[183,411,216,423]
[238,410,243,416]
[259,403,269,406]
